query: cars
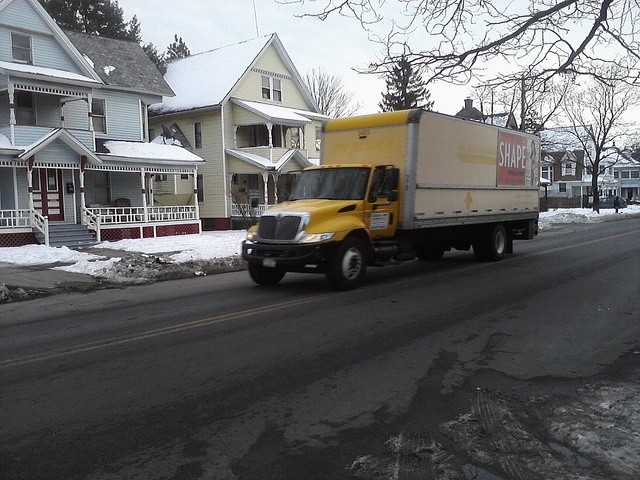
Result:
[590,195,627,208]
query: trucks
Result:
[240,107,541,289]
